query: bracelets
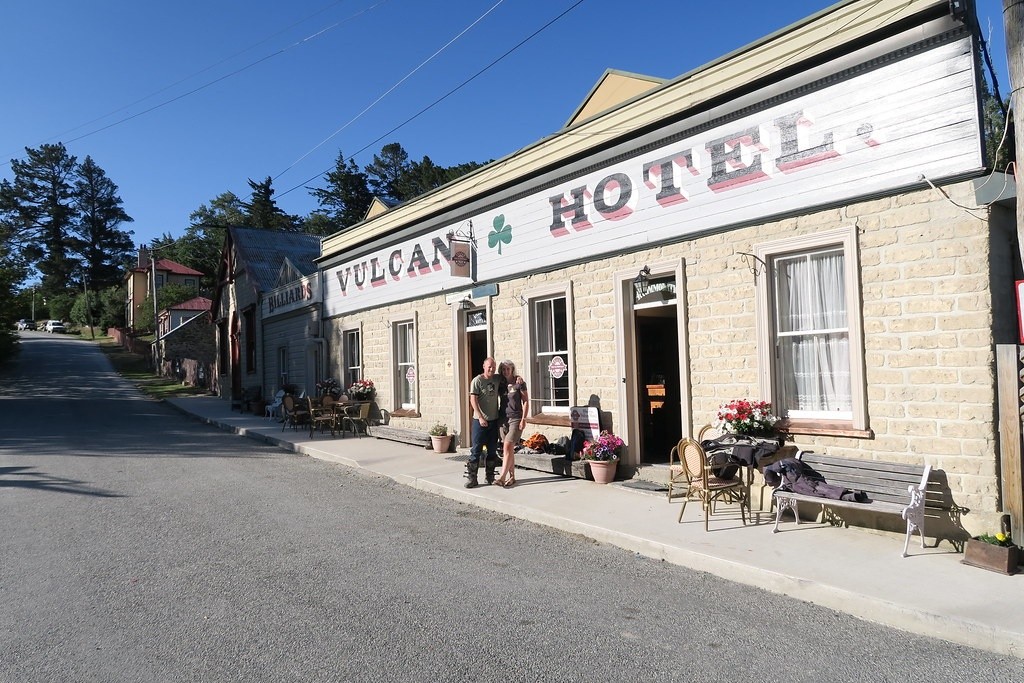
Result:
[522,418,526,421]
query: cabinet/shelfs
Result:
[646,384,667,426]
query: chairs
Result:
[668,424,728,502]
[264,389,351,440]
[678,437,746,531]
[228,387,247,414]
[342,402,373,439]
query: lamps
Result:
[633,265,650,295]
[458,294,471,311]
[233,330,241,343]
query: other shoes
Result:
[464,479,478,488]
[483,478,496,485]
[505,478,516,486]
[491,479,505,487]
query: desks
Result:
[704,436,795,525]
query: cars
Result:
[18,319,37,331]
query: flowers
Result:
[578,430,625,461]
[346,380,373,395]
[978,532,1014,547]
[710,399,782,433]
[316,378,341,395]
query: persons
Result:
[494,358,529,488]
[464,357,523,488]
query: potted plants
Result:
[429,421,454,453]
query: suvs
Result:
[38,319,66,334]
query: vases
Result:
[586,458,620,483]
[355,392,368,401]
[736,429,773,439]
[961,536,1022,576]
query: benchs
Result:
[770,450,933,558]
[499,422,590,479]
[365,409,432,448]
